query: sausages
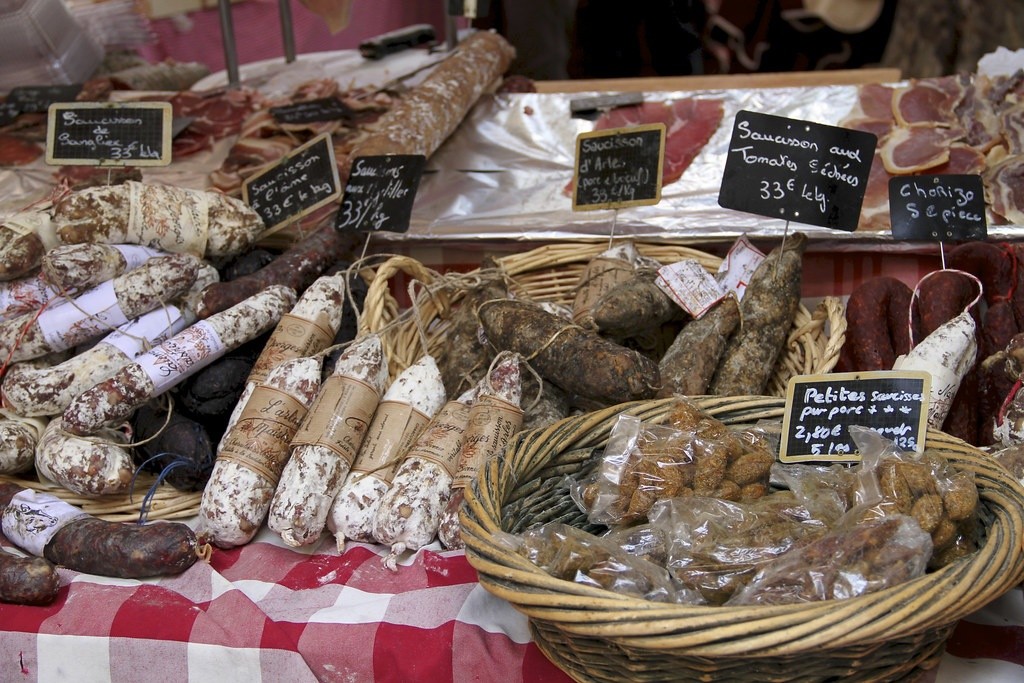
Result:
[0,180,1024,609]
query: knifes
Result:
[199,24,437,97]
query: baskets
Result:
[458,395,1024,683]
[0,263,395,527]
[358,242,848,434]
